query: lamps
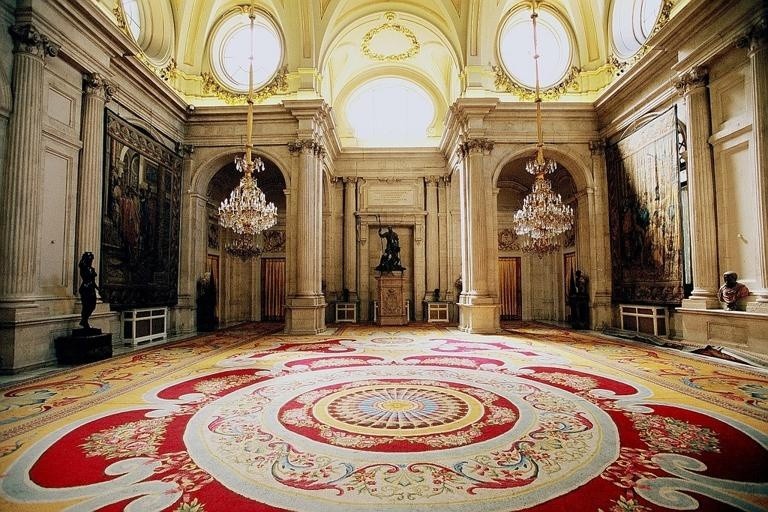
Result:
[520,235,561,260]
[508,1,580,257]
[216,232,261,261]
[211,0,291,236]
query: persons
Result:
[719,271,749,303]
[575,270,590,294]
[78,252,101,327]
[377,225,400,270]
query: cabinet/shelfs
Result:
[421,300,451,324]
[119,305,169,346]
[333,302,359,324]
[617,301,671,340]
[372,299,411,324]
